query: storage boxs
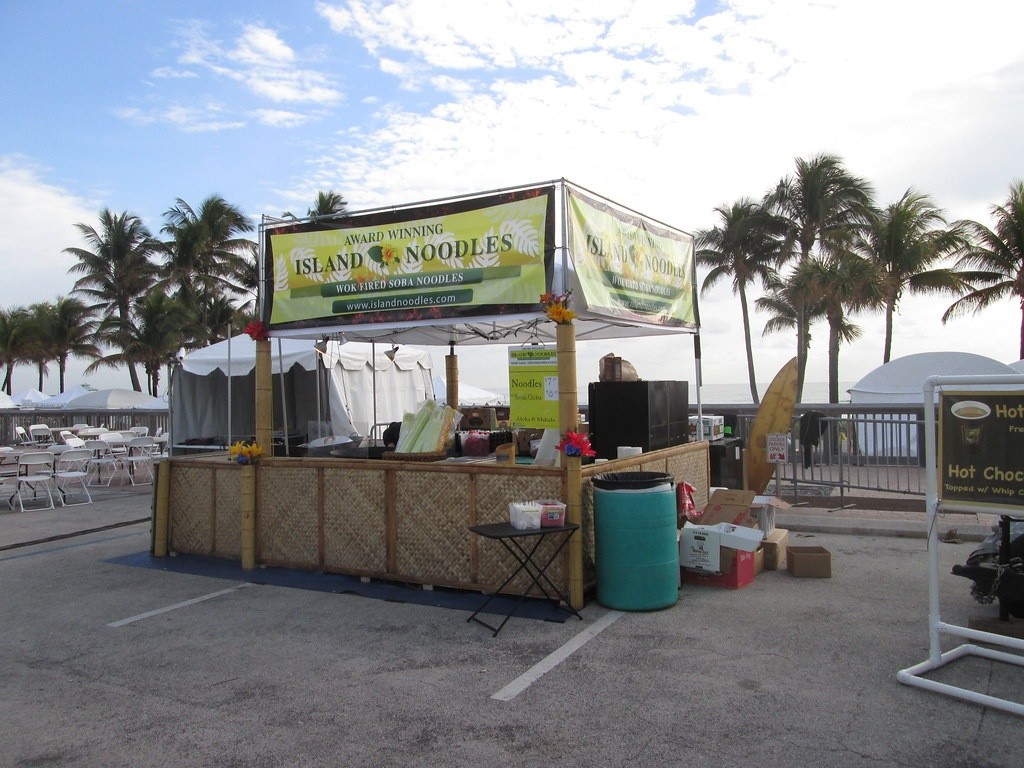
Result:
[786,545,833,578]
[532,500,567,527]
[508,500,543,530]
[676,487,789,590]
[688,415,725,442]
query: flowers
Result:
[226,440,264,464]
[539,285,585,325]
[243,319,271,342]
[554,430,599,458]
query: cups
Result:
[951,401,991,452]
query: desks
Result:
[466,519,585,639]
[50,427,81,443]
[95,440,135,485]
[0,452,66,511]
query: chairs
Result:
[0,423,171,514]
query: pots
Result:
[330,447,395,459]
[296,435,364,456]
